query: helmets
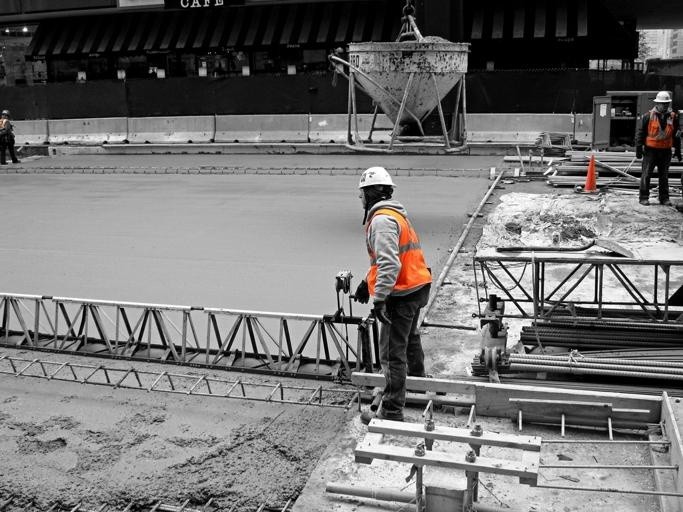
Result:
[2,110,10,115]
[653,92,672,103]
[358,167,397,188]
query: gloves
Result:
[353,280,370,304]
[636,146,644,159]
[374,300,392,325]
[672,149,682,162]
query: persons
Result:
[0,109,20,165]
[353,166,432,425]
[634,90,682,206]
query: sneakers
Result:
[639,200,649,206]
[361,411,403,424]
[2,159,22,165]
[661,201,673,205]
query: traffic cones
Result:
[582,154,601,193]
